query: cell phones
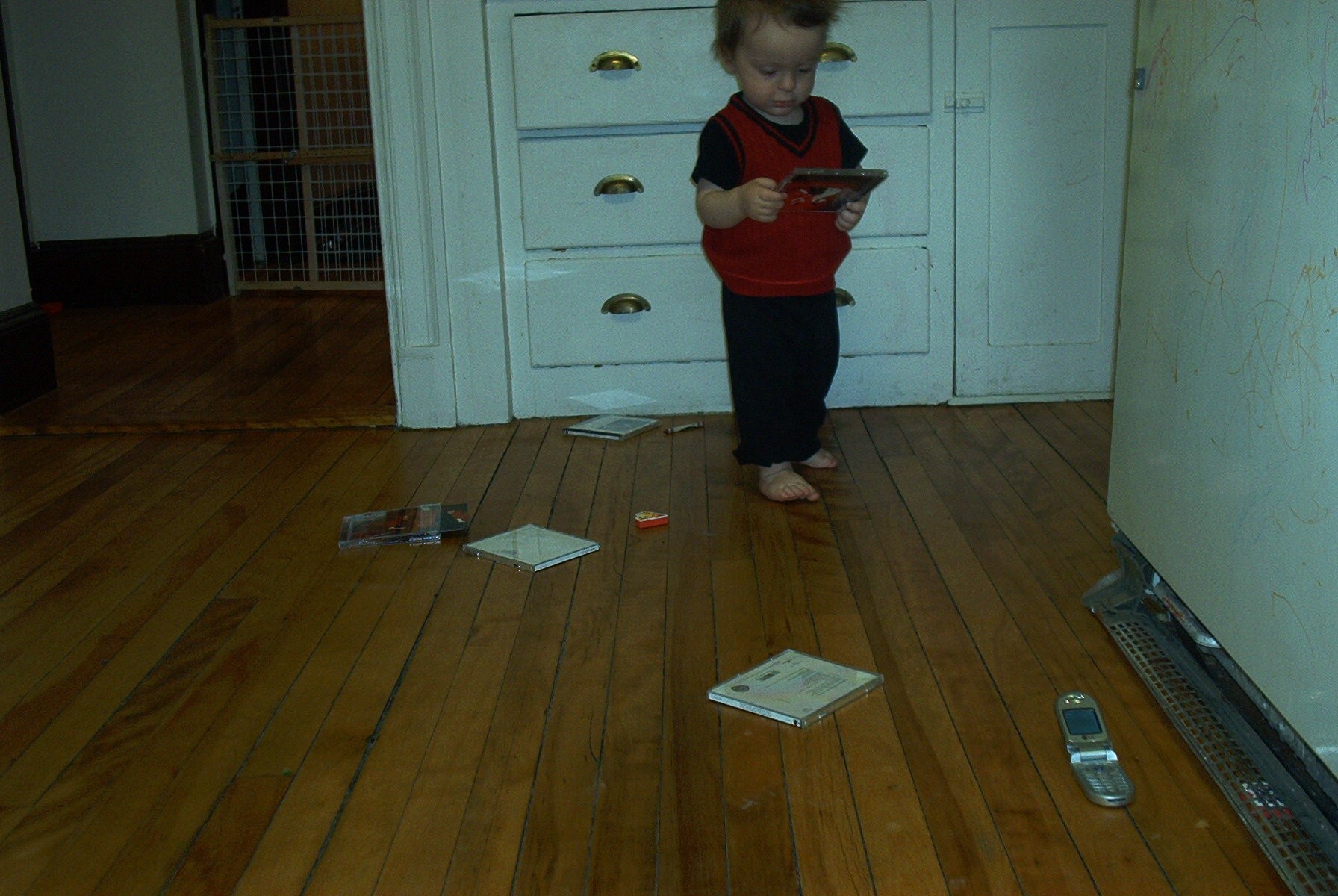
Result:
[1055,690,1138,808]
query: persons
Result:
[688,0,879,508]
